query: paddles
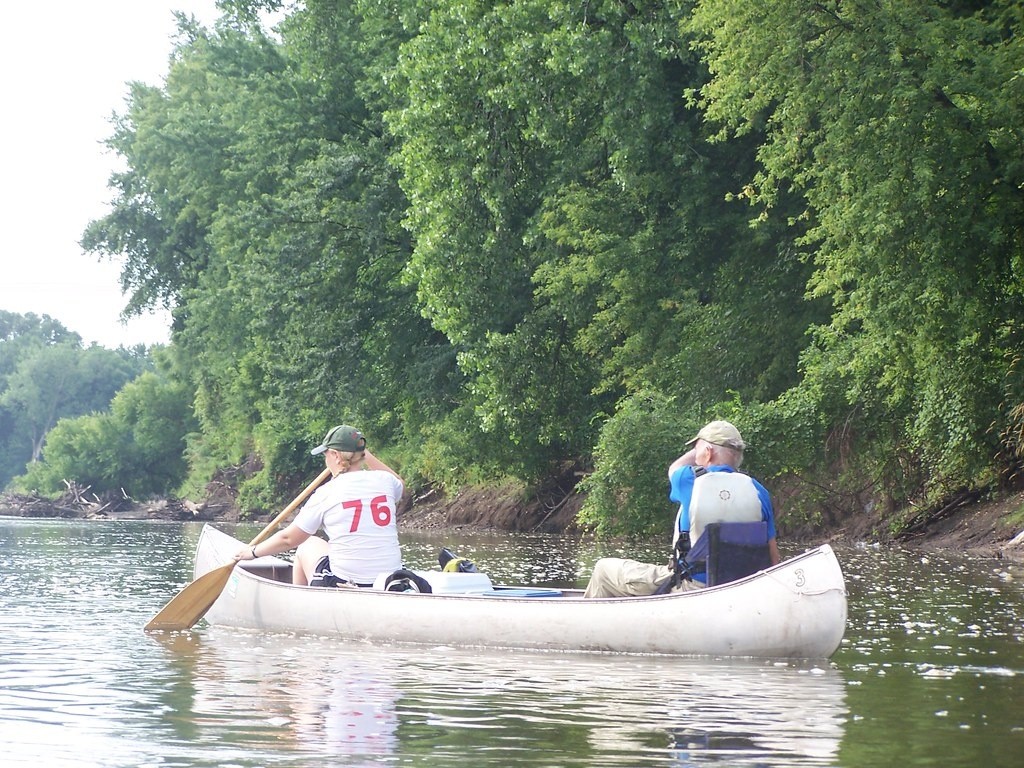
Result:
[141,466,337,635]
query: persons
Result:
[583,421,780,599]
[234,425,405,587]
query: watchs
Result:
[251,545,259,558]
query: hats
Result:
[684,420,743,451]
[311,425,366,455]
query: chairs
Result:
[653,522,770,594]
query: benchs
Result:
[373,571,494,593]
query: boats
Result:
[189,520,848,664]
